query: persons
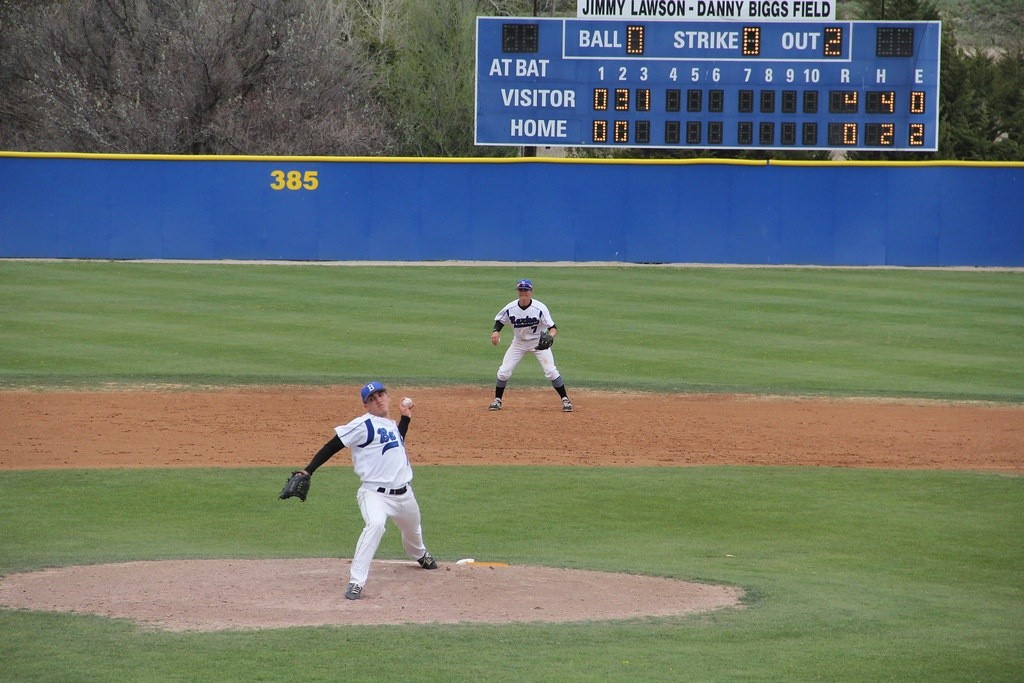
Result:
[488,279,573,412]
[279,381,438,600]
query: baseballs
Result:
[402,398,412,408]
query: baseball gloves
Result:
[534,330,554,351]
[277,470,312,502]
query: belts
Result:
[376,485,408,495]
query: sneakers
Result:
[561,399,573,412]
[488,398,502,411]
[344,583,363,601]
[417,551,438,570]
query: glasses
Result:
[518,289,530,292]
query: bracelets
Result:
[493,330,497,332]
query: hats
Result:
[515,279,533,291]
[360,381,387,404]
